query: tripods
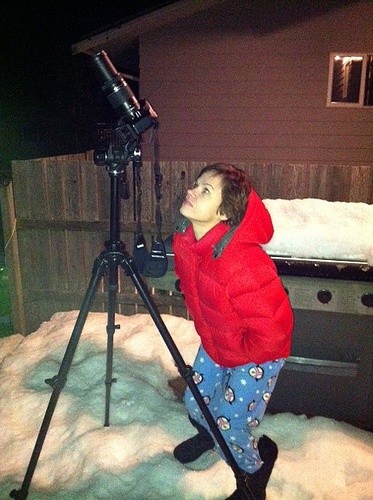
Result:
[10,140,256,500]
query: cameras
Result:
[87,51,158,144]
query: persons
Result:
[173,162,294,500]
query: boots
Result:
[224,435,278,500]
[174,413,216,465]
